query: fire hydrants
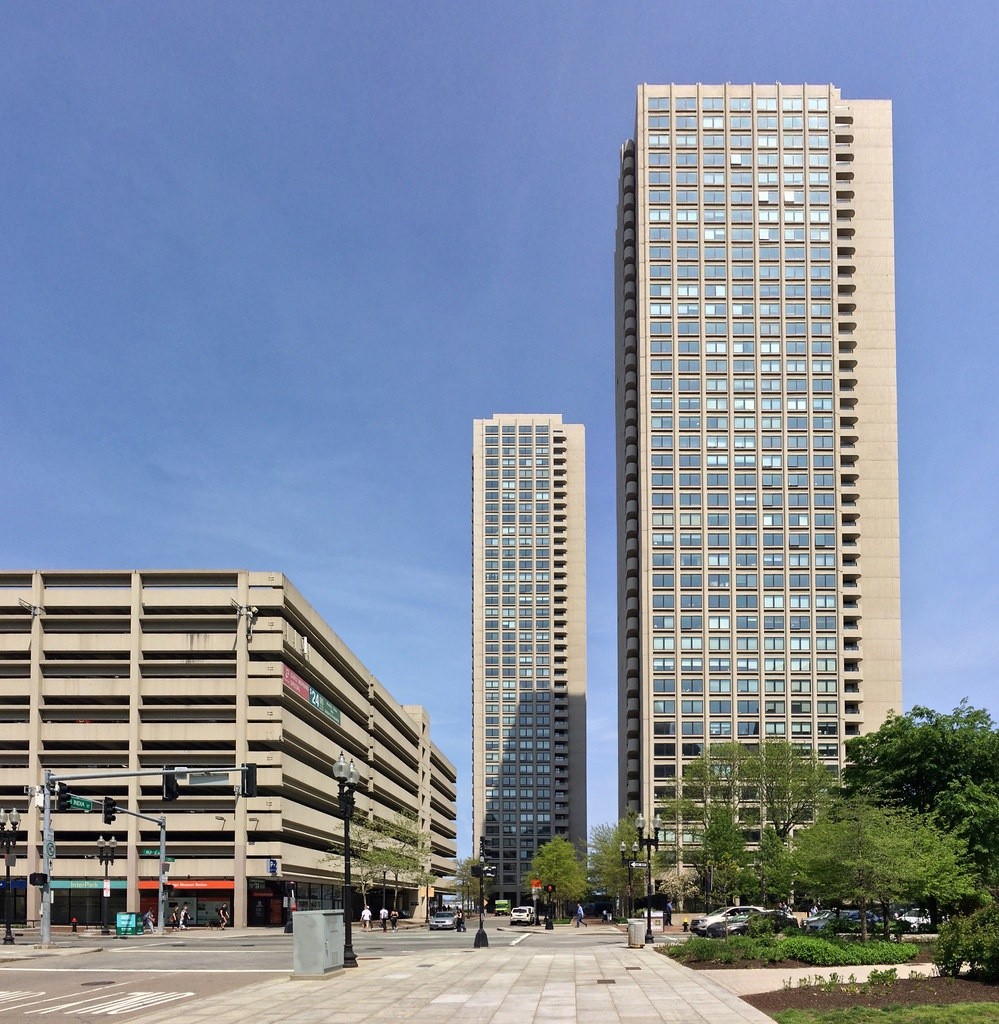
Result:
[682,917,689,932]
[71,917,78,932]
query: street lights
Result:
[473,841,491,948]
[330,751,364,969]
[382,863,388,909]
[635,812,662,946]
[0,807,21,945]
[96,834,118,936]
[619,840,640,932]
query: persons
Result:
[575,903,587,928]
[437,905,450,912]
[181,906,190,929]
[811,905,818,915]
[779,902,787,910]
[456,908,466,932]
[602,910,606,922]
[220,903,229,929]
[380,906,388,932]
[390,908,398,932]
[362,906,373,928]
[172,906,180,931]
[665,901,675,926]
[144,909,156,932]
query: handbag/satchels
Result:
[169,916,174,922]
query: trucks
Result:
[494,900,512,916]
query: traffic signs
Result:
[629,861,648,868]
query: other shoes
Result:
[396,927,398,929]
[391,930,394,932]
[664,924,668,925]
[575,926,579,928]
[222,928,224,930]
[384,929,387,932]
[670,924,673,926]
[585,922,587,927]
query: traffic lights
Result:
[543,884,556,893]
[103,796,117,825]
[162,765,178,803]
[29,872,48,887]
[54,781,72,812]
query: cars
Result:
[705,911,798,938]
[519,906,534,923]
[800,908,947,935]
[429,911,457,930]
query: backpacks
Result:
[383,912,388,919]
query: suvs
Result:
[689,905,768,937]
[510,907,534,926]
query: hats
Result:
[366,906,369,908]
